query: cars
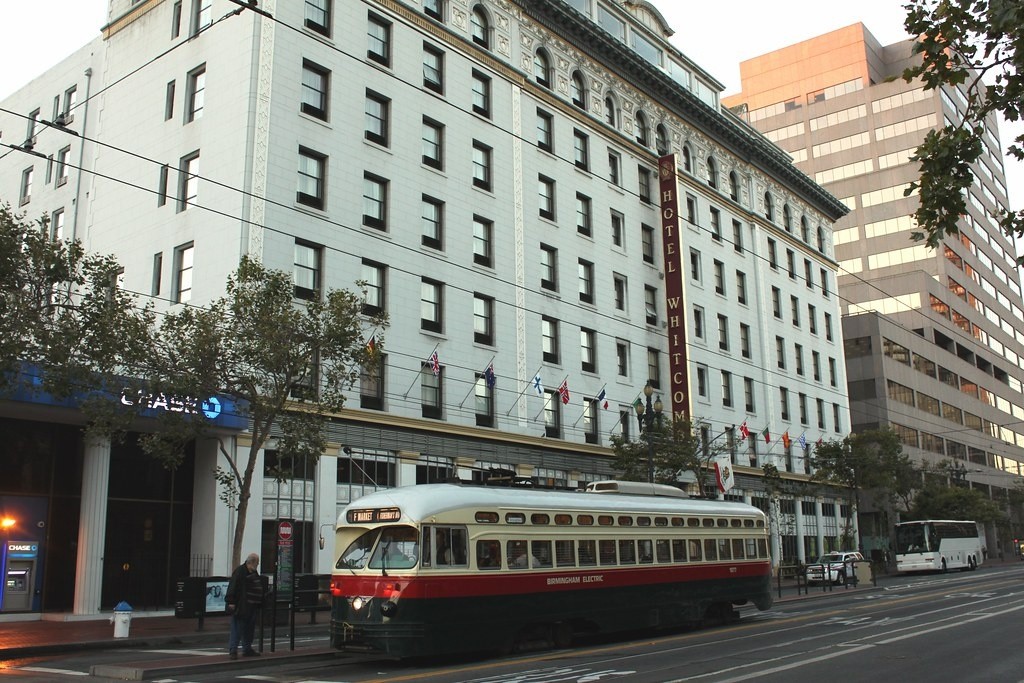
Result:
[806,550,866,585]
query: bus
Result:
[318,480,774,660]
[894,520,984,574]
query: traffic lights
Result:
[1014,539,1018,543]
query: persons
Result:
[427,531,455,565]
[206,586,215,604]
[480,544,498,568]
[907,542,920,551]
[226,553,264,660]
[378,531,409,562]
[215,586,223,603]
[509,543,541,567]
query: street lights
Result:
[842,432,868,556]
[636,379,664,483]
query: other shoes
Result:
[230,650,237,659]
[243,651,261,657]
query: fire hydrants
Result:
[108,600,133,638]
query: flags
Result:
[365,336,376,355]
[633,396,645,416]
[483,365,496,390]
[714,452,735,493]
[799,432,806,451]
[740,421,749,440]
[428,352,440,377]
[559,379,570,405]
[532,373,544,396]
[817,438,822,445]
[598,388,608,410]
[761,427,770,444]
[781,431,790,449]
